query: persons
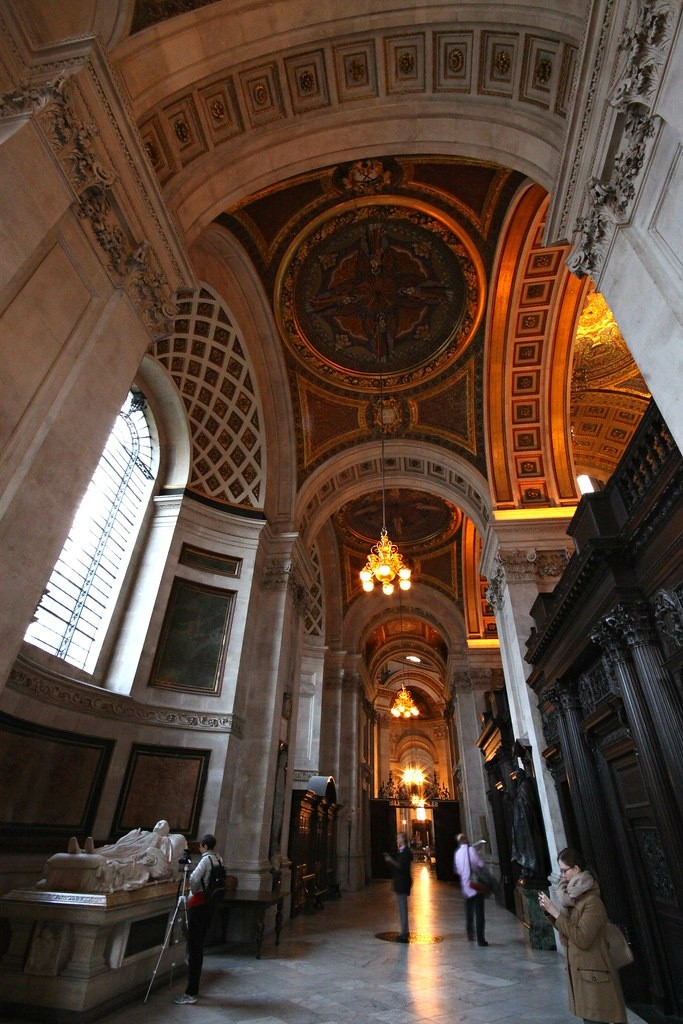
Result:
[383,831,412,944]
[454,834,490,947]
[537,848,628,1024]
[173,835,224,1004]
[67,820,173,869]
[508,768,543,879]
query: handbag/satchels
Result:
[469,871,491,899]
[605,924,633,968]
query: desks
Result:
[222,890,291,960]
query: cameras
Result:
[178,857,191,865]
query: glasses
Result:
[560,867,575,874]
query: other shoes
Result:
[468,936,475,941]
[478,940,488,946]
[396,935,409,943]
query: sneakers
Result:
[174,993,197,1004]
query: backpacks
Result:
[199,856,227,906]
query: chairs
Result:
[302,870,344,911]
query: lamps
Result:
[359,357,412,595]
[391,591,419,717]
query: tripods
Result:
[143,864,189,1005]
